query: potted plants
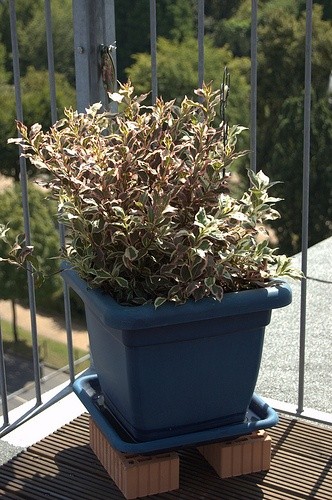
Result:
[0,68,307,440]
